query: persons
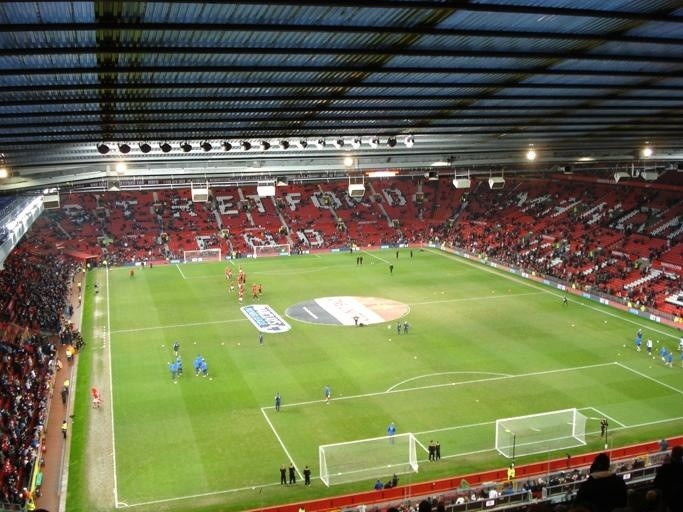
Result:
[396,322,401,336]
[323,385,330,405]
[258,331,263,345]
[352,316,359,327]
[0,193,102,512]
[277,415,683,512]
[274,393,281,412]
[402,321,410,335]
[633,328,683,368]
[61,174,682,320]
[165,342,208,384]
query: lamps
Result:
[613,160,658,183]
[94,132,416,155]
[107,176,120,191]
[41,188,60,209]
